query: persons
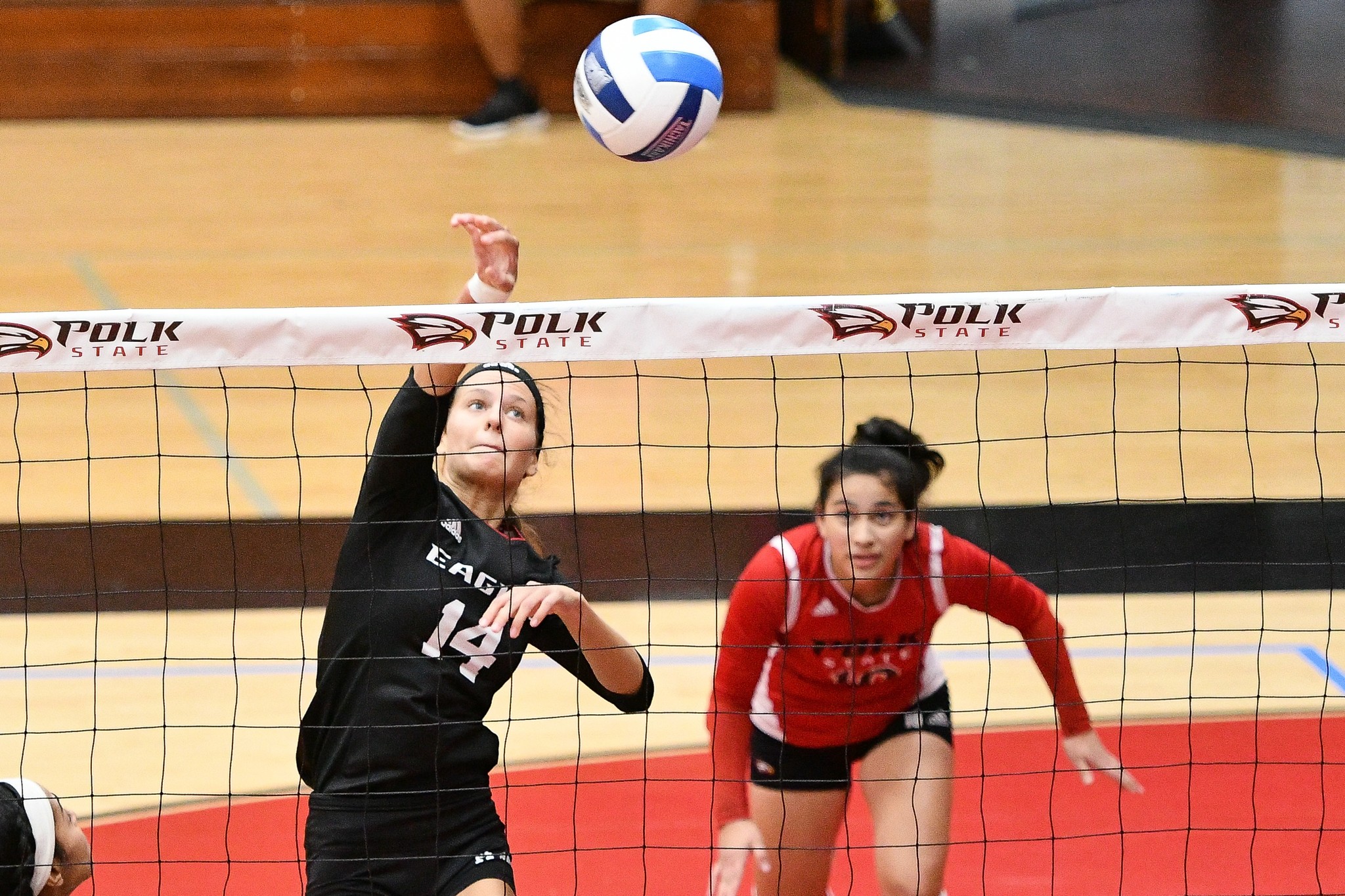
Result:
[447,1,701,141]
[294,213,654,896]
[707,415,1149,895]
[0,784,97,896]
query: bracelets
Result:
[468,271,511,307]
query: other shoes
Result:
[453,79,550,137]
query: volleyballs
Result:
[573,14,724,164]
